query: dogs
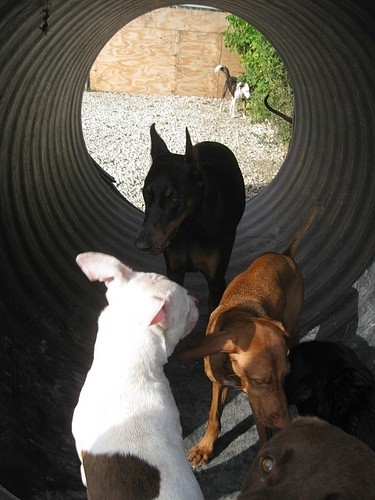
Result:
[214,64,251,120]
[238,340,374,500]
[72,252,203,499]
[176,203,318,469]
[136,124,247,310]
